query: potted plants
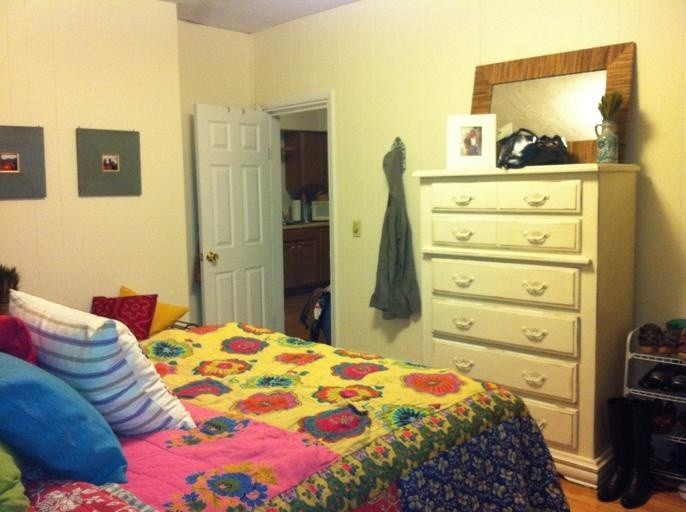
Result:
[0,264,21,314]
[595,91,624,162]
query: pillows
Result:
[0,350,128,483]
[0,439,33,512]
[0,315,37,365]
[91,293,158,340]
[120,286,190,334]
[10,289,198,432]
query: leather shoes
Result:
[652,402,677,433]
[661,368,685,394]
[671,411,686,437]
[639,364,673,388]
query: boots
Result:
[596,397,624,502]
[620,399,652,508]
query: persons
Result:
[1,158,17,170]
[465,129,479,156]
[108,158,117,170]
[104,157,109,170]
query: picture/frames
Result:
[0,125,47,200]
[446,114,496,174]
[75,128,143,197]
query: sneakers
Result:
[675,328,686,357]
[638,324,662,354]
[658,329,679,354]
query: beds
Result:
[0,289,571,512]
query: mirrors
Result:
[469,41,636,162]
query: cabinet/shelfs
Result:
[280,128,327,200]
[282,226,332,296]
[622,320,686,502]
[411,162,642,491]
